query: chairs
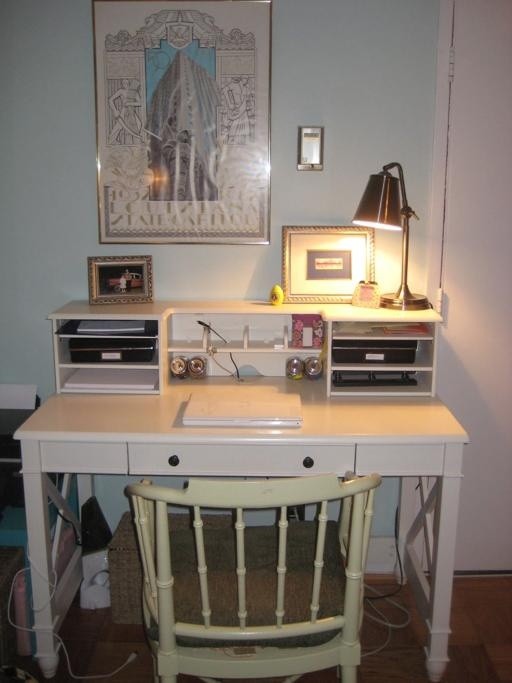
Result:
[123,470,383,683]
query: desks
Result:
[13,395,470,683]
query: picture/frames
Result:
[282,226,375,304]
[87,255,153,305]
[92,1,272,246]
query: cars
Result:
[107,273,144,292]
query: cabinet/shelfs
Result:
[44,300,444,397]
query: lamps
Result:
[351,162,430,311]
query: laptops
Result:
[182,386,304,427]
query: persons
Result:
[119,269,132,293]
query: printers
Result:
[1,390,64,516]
